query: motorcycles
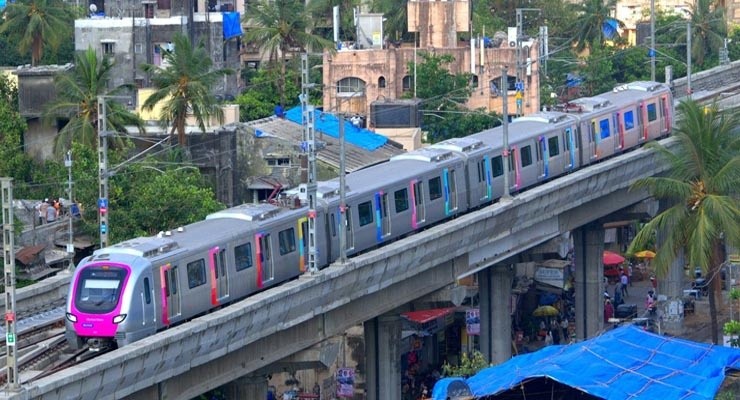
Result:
[614,302,639,319]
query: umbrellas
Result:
[634,250,657,264]
[533,305,559,315]
[603,250,625,265]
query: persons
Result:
[345,113,366,128]
[401,350,441,400]
[336,369,354,384]
[603,262,657,324]
[34,197,62,226]
[542,316,569,348]
[277,380,320,400]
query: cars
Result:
[683,289,702,301]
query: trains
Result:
[65,80,676,365]
